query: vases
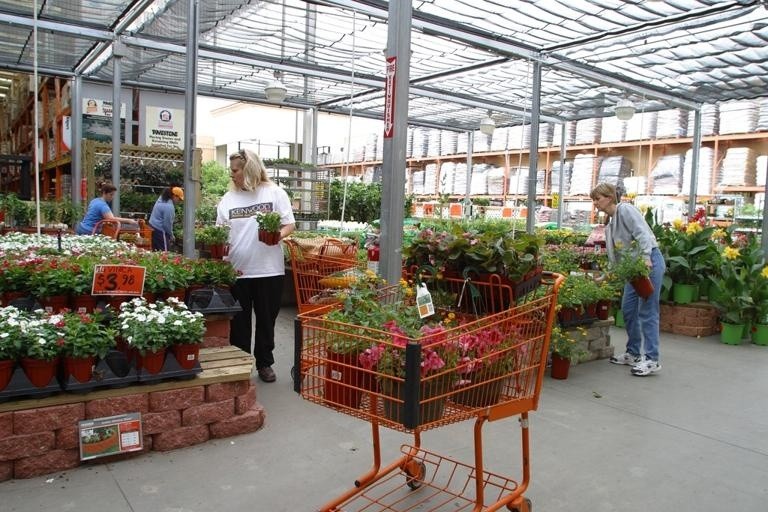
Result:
[106,350,136,378]
[21,358,60,387]
[323,349,366,410]
[381,375,450,429]
[139,344,170,375]
[171,342,200,369]
[449,368,509,408]
[549,357,569,379]
[63,356,97,383]
[1,284,237,311]
[1,360,19,390]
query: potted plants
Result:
[256,212,282,246]
[327,179,414,221]
[282,218,614,329]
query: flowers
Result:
[163,297,207,346]
[322,278,531,385]
[0,306,28,360]
[116,297,170,353]
[67,304,116,357]
[0,221,243,297]
[615,189,768,346]
[19,305,69,359]
[550,327,588,361]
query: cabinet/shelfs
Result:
[318,160,383,185]
[470,149,531,205]
[695,134,767,215]
[0,69,204,213]
[536,137,694,216]
[404,154,470,203]
[263,162,333,232]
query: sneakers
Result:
[257,365,276,383]
[630,358,663,377]
[609,351,643,366]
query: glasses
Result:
[238,149,247,163]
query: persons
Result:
[76,184,116,235]
[148,187,184,250]
[215,149,296,382]
[591,182,666,376]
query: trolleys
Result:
[283,236,366,390]
[295,269,565,511]
[91,219,121,242]
[103,219,153,251]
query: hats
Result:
[171,186,184,201]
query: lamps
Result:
[480,110,498,138]
[263,71,289,106]
[614,89,639,121]
